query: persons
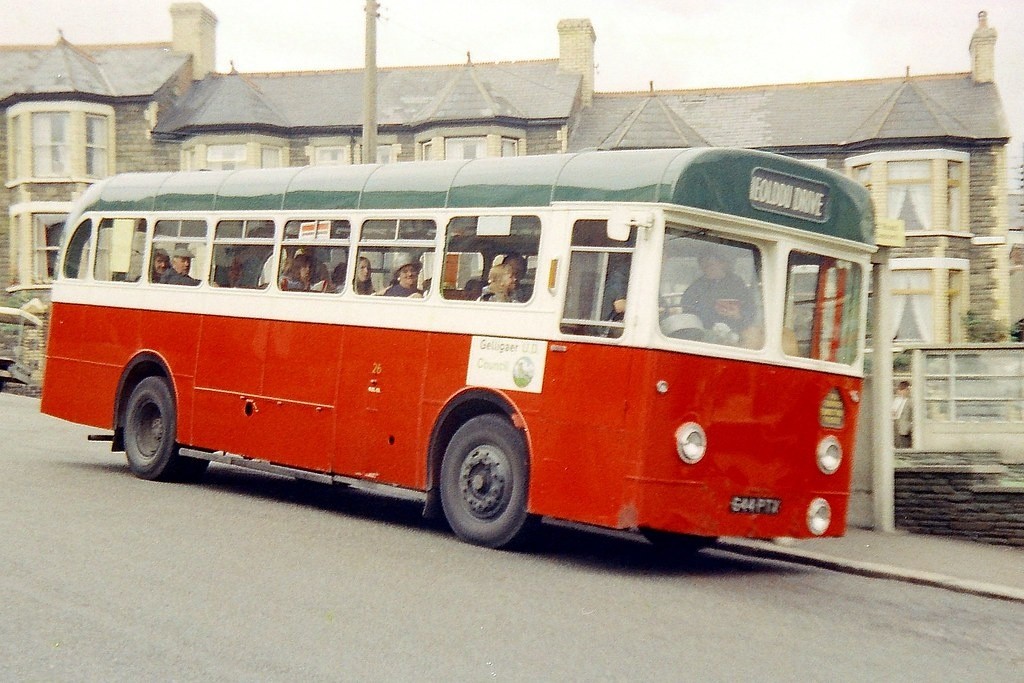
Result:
[602,262,663,322]
[383,255,425,297]
[338,257,375,295]
[231,225,346,292]
[682,251,757,329]
[475,254,532,304]
[151,248,200,285]
[894,381,913,449]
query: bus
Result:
[36,144,878,550]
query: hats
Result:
[171,248,195,258]
[391,253,422,276]
